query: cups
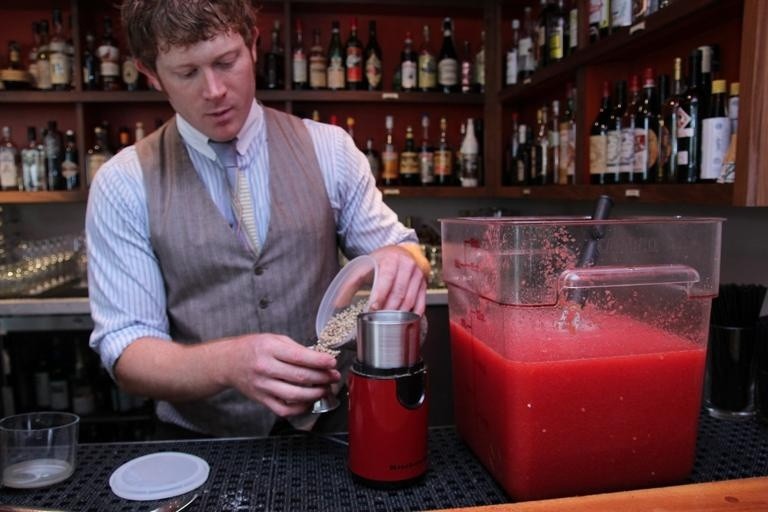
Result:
[0,411,80,489]
[421,244,446,287]
[315,255,428,350]
[704,321,762,423]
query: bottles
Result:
[0,0,739,192]
[0,336,155,418]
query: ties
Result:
[208,137,261,259]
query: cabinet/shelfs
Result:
[493,0,768,208]
[0,1,500,203]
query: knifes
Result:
[149,489,202,512]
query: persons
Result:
[83,0,431,439]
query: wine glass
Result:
[1,232,87,296]
